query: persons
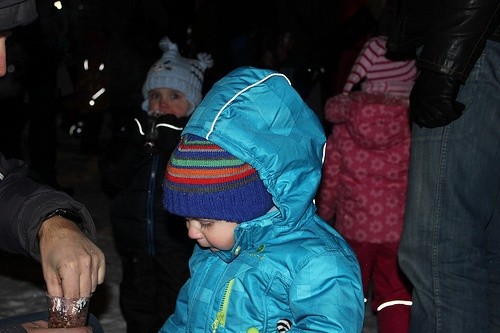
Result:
[385,0,500,333]
[110,36,213,333]
[321,35,416,333]
[158,67,365,333]
[0,0,106,333]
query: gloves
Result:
[408,69,466,129]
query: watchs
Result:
[45,208,84,225]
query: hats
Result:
[163,132,274,223]
[140,38,213,117]
[342,36,417,104]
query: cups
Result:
[45,291,92,328]
